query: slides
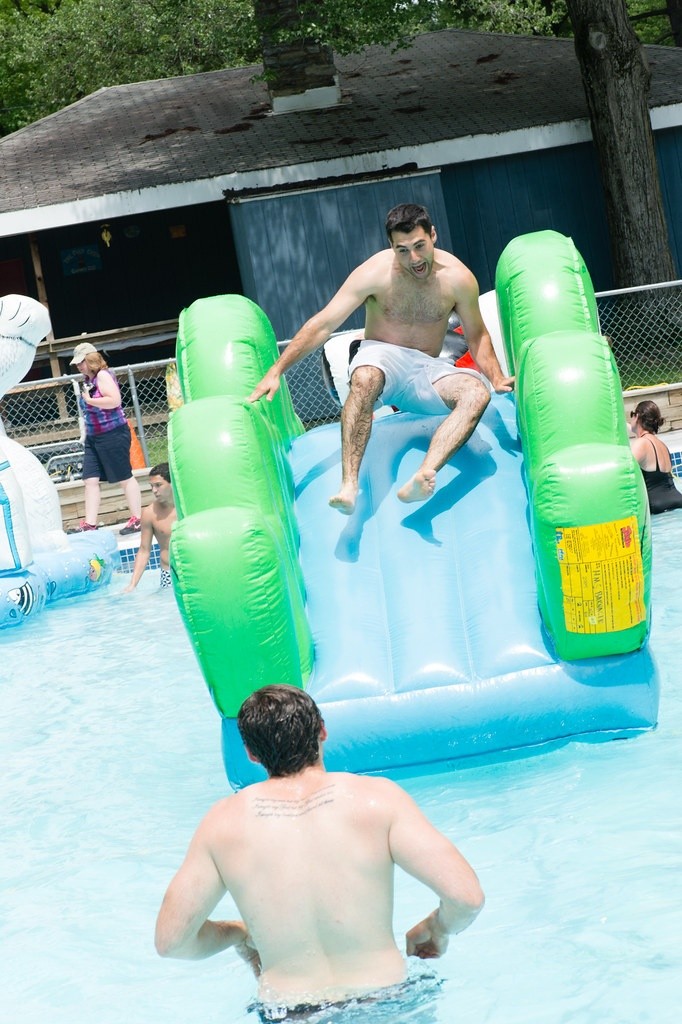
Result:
[167,225,663,785]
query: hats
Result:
[69,342,96,366]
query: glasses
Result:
[630,411,636,418]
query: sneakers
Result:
[67,522,98,535]
[119,516,141,535]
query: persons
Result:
[154,683,484,1003]
[246,203,515,516]
[67,342,142,536]
[124,462,177,590]
[628,399,682,515]
